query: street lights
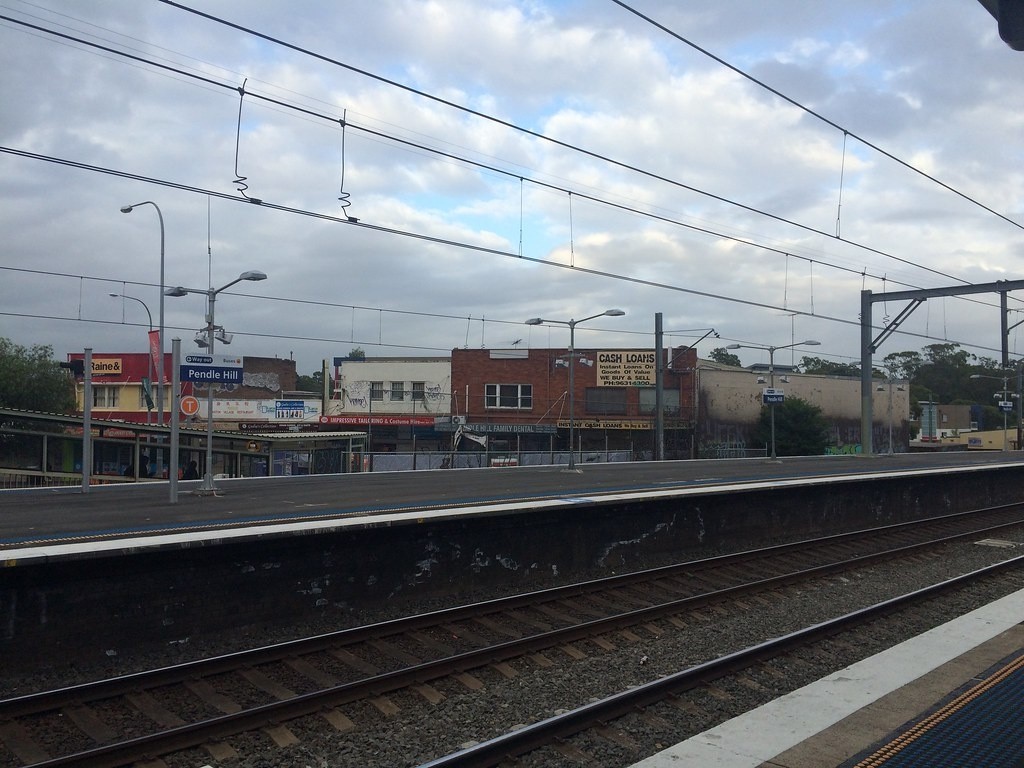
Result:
[164,270,267,490]
[526,310,625,473]
[120,201,165,478]
[876,380,905,453]
[726,341,821,461]
[110,293,152,459]
[971,374,1024,452]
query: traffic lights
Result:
[59,359,84,377]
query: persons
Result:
[181,460,199,480]
[139,456,154,478]
